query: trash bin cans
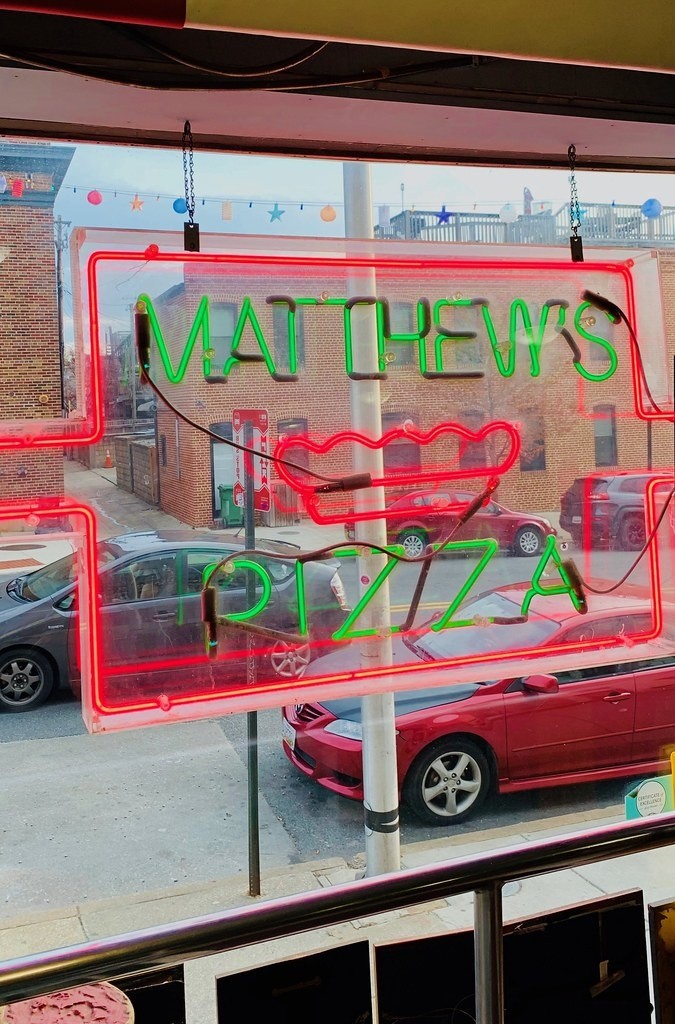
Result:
[218,484,244,529]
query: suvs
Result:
[559,472,674,552]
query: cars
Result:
[2,526,351,715]
[344,489,558,561]
[278,578,674,827]
